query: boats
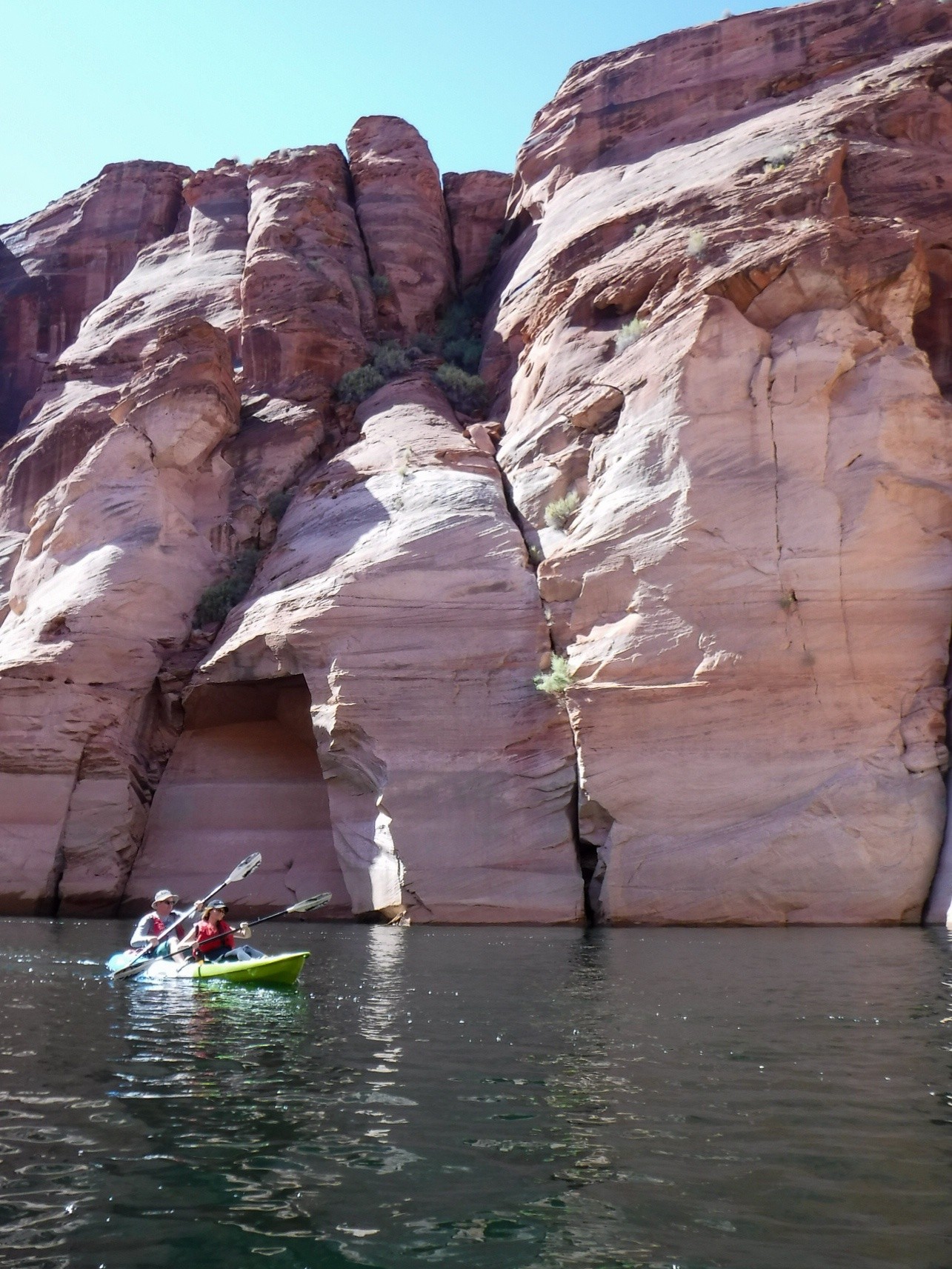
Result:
[102,949,313,986]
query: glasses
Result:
[160,901,175,905]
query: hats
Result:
[151,890,179,910]
[206,899,229,913]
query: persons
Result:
[130,889,203,963]
[177,899,266,964]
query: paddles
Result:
[109,852,263,978]
[113,892,333,982]
